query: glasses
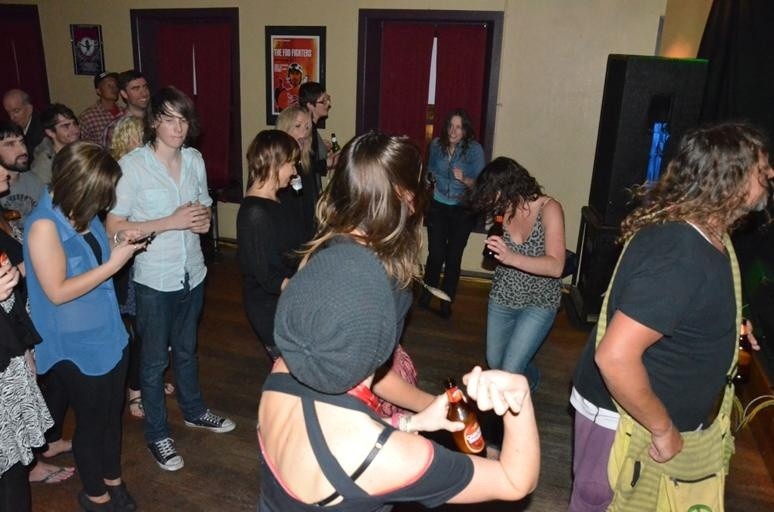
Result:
[315,94,330,104]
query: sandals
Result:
[164,382,176,395]
[128,394,146,420]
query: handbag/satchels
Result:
[605,416,727,512]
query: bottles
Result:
[290,168,306,196]
[331,132,340,154]
[734,318,752,384]
[481,205,506,271]
[0,249,19,282]
[442,377,487,458]
[427,172,435,196]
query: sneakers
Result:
[439,296,453,316]
[416,291,433,307]
[183,408,237,435]
[146,436,185,473]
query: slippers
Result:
[38,441,72,463]
[29,464,80,487]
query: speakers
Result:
[563,206,622,331]
[589,53,708,228]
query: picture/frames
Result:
[265,25,327,126]
[69,23,105,77]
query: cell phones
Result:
[128,231,155,244]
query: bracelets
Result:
[391,411,413,435]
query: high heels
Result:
[105,481,137,512]
[75,489,123,512]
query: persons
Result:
[456,156,567,394]
[295,127,438,435]
[418,106,485,318]
[278,61,308,110]
[0,70,236,512]
[567,117,774,512]
[255,235,541,512]
[236,81,340,364]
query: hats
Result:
[91,70,120,85]
[272,241,399,396]
